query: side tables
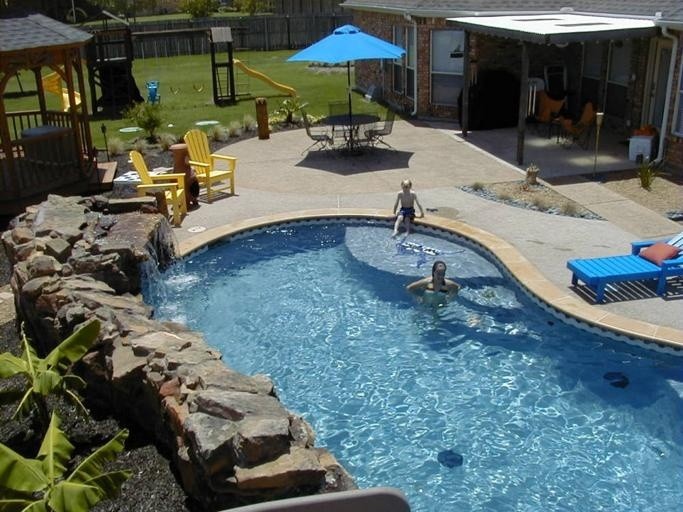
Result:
[114,171,167,197]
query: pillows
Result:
[640,242,683,265]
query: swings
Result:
[164,39,181,95]
[187,38,205,92]
[140,40,160,106]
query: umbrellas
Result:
[285,23,406,151]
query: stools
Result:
[627,136,654,162]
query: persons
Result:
[187,166,200,207]
[406,260,460,305]
[392,179,425,240]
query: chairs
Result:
[129,150,187,223]
[301,102,396,159]
[527,90,595,151]
[567,234,683,303]
[183,128,237,202]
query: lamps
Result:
[449,44,464,59]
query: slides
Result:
[233,60,296,97]
[42,65,81,113]
